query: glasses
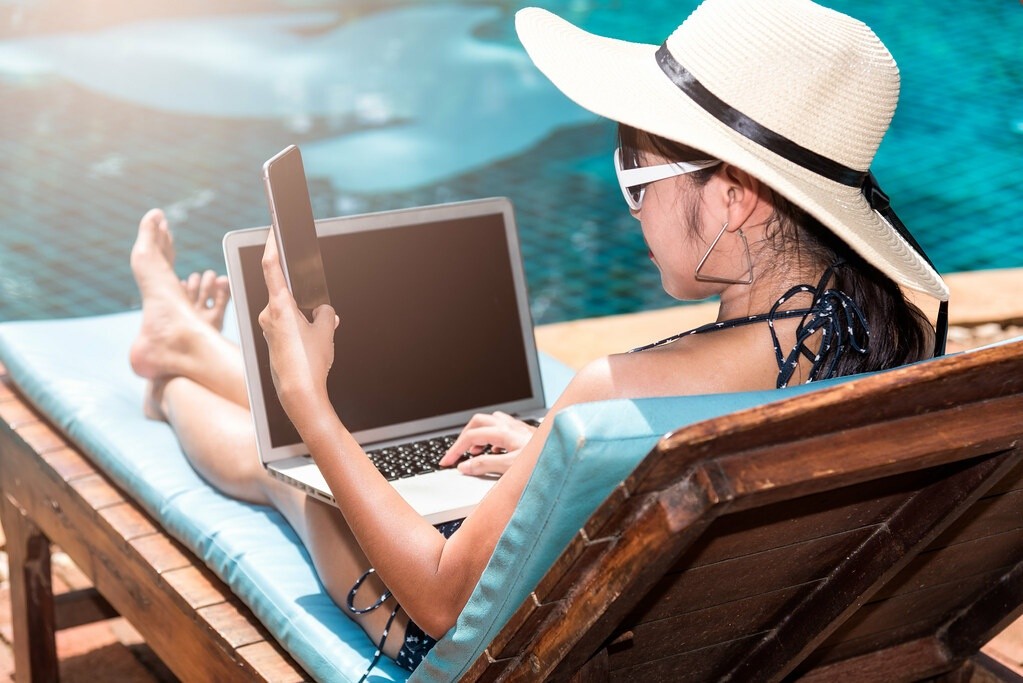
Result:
[613,145,722,212]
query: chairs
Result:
[0,299,1023,683]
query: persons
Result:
[128,0,950,672]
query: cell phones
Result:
[265,143,338,324]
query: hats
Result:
[516,0,952,304]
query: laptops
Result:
[221,196,549,534]
